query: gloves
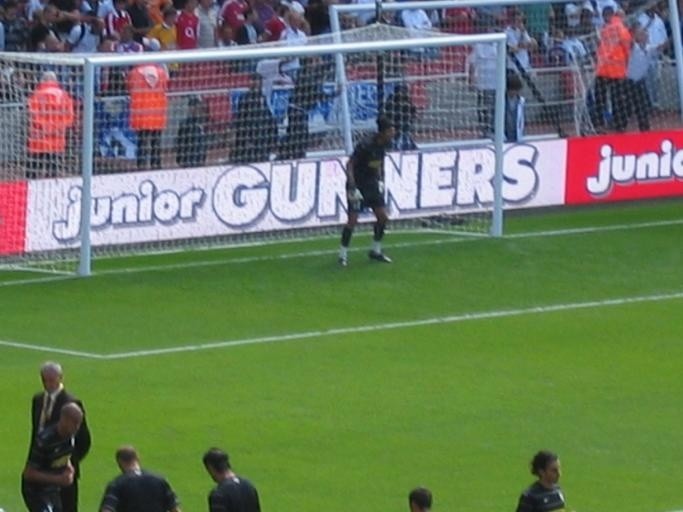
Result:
[347,188,364,212]
[378,180,387,196]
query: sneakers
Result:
[336,248,349,266]
[368,248,392,263]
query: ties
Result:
[44,395,52,413]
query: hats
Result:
[375,113,394,128]
[141,36,162,52]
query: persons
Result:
[409,487,432,512]
[99,446,181,512]
[1,0,682,179]
[22,362,91,512]
[338,120,396,266]
[516,450,568,512]
[203,447,261,512]
